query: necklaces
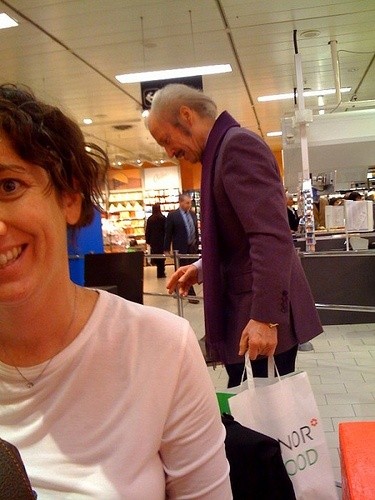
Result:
[5,284,78,388]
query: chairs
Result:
[220,413,297,500]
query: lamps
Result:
[114,10,351,137]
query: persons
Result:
[163,194,200,304]
[313,190,375,230]
[0,81,234,500]
[145,204,169,278]
[284,193,299,232]
[145,84,324,388]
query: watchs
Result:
[266,322,279,328]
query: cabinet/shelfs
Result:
[100,188,203,254]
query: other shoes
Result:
[157,272,165,279]
[189,293,199,303]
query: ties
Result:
[186,211,195,246]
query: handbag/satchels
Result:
[216,349,339,500]
